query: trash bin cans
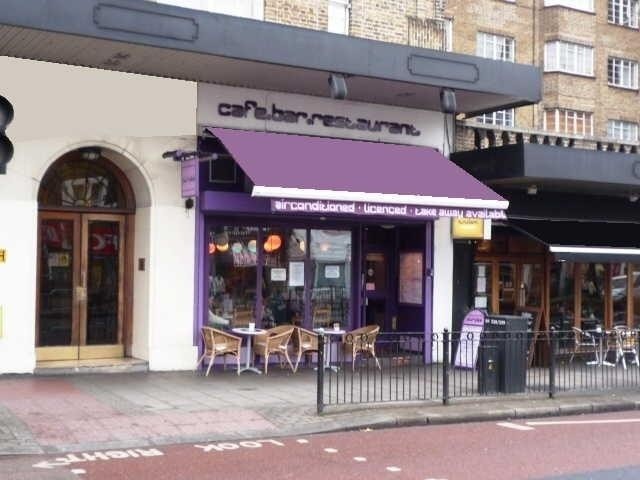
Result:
[477,315,528,394]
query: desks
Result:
[313,329,345,373]
[233,328,267,375]
[587,329,617,366]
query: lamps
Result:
[527,183,537,195]
[440,88,457,113]
[629,190,639,202]
[327,72,348,100]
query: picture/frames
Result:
[397,248,425,308]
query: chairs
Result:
[293,304,332,331]
[569,326,599,364]
[252,324,295,376]
[603,326,639,370]
[291,324,326,373]
[340,326,382,372]
[194,326,242,377]
